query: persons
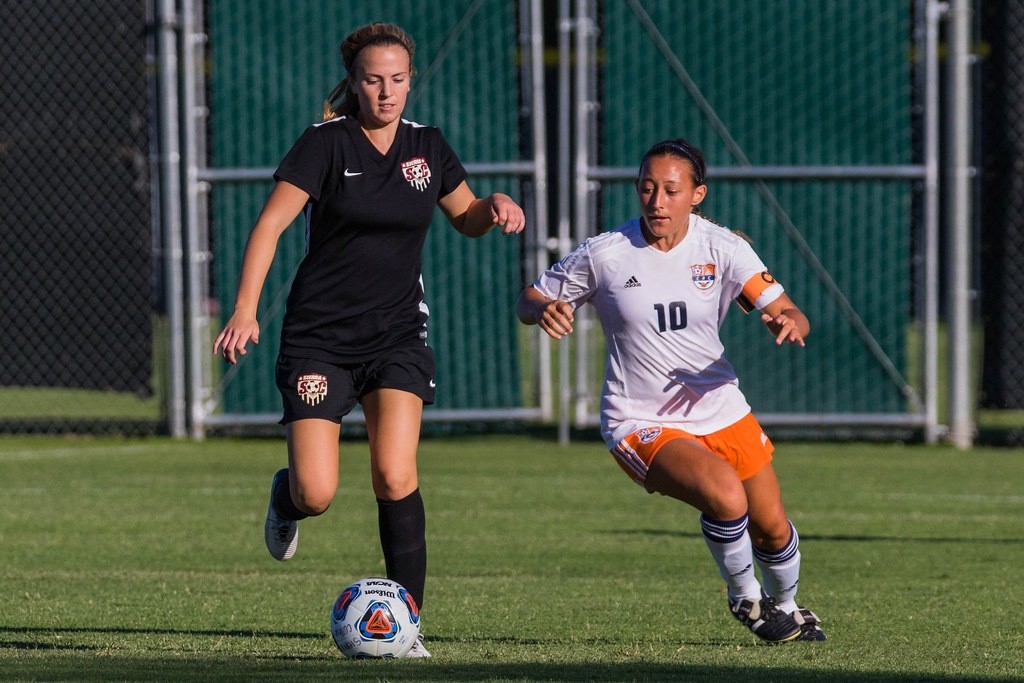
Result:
[209,23,526,661]
[517,137,830,644]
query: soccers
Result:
[330,577,421,658]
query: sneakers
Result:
[728,583,801,643]
[761,585,827,641]
[265,467,301,561]
[405,631,433,659]
